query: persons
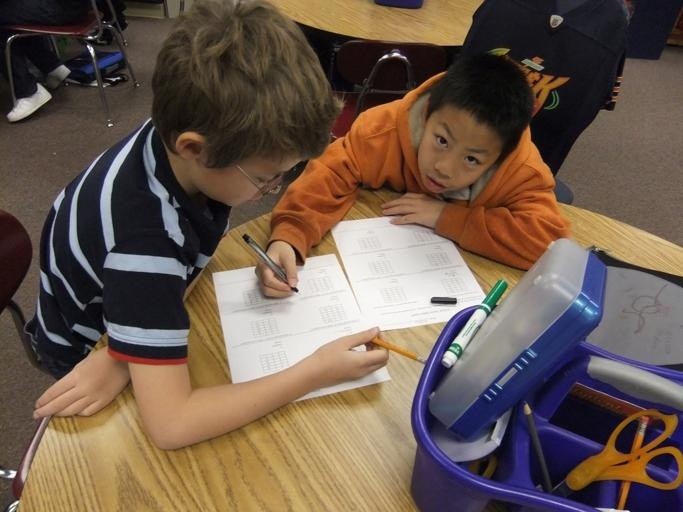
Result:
[0,1,95,123]
[255,52,573,301]
[23,1,390,451]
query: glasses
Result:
[231,164,288,195]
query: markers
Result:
[441,280,508,368]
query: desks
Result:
[263,0,486,47]
[17,190,683,512]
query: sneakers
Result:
[43,63,72,91]
[6,82,53,124]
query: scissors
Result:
[468,453,498,480]
[551,408,683,500]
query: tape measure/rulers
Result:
[570,380,656,426]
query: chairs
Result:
[0,0,185,128]
[335,40,450,139]
[0,208,52,512]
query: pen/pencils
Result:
[243,233,303,295]
[371,336,429,365]
[616,415,649,510]
[522,400,552,494]
